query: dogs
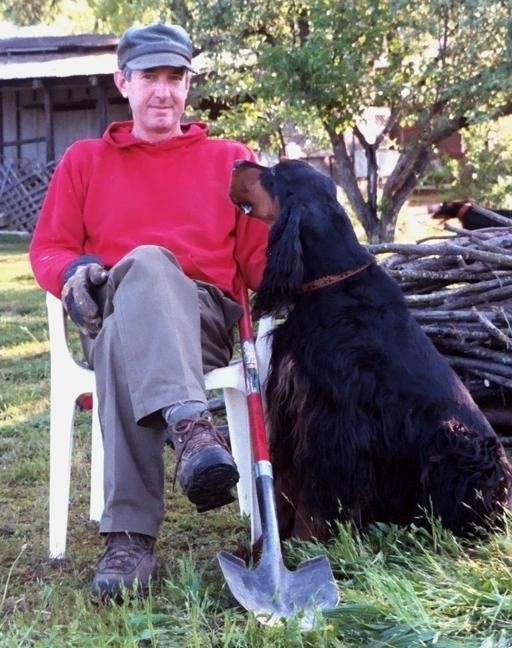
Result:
[225,156,511,564]
[433,197,511,231]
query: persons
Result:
[27,20,272,606]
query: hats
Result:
[117,24,198,74]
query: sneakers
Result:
[92,532,156,605]
[166,409,239,512]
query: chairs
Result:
[45,290,276,557]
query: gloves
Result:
[61,256,110,338]
